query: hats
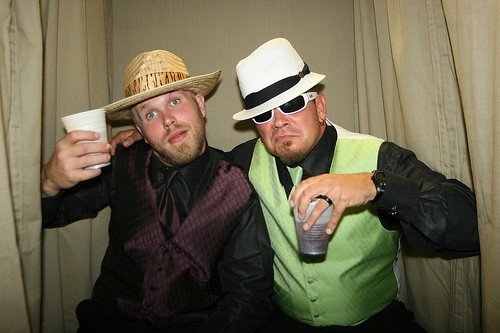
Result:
[102,49,223,120]
[232,35,328,123]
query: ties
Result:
[157,166,178,236]
[286,165,305,204]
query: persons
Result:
[108,38,480,333]
[40,49,276,333]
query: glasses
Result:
[251,91,317,125]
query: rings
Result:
[317,196,333,206]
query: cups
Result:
[293,198,334,256]
[62,109,111,170]
